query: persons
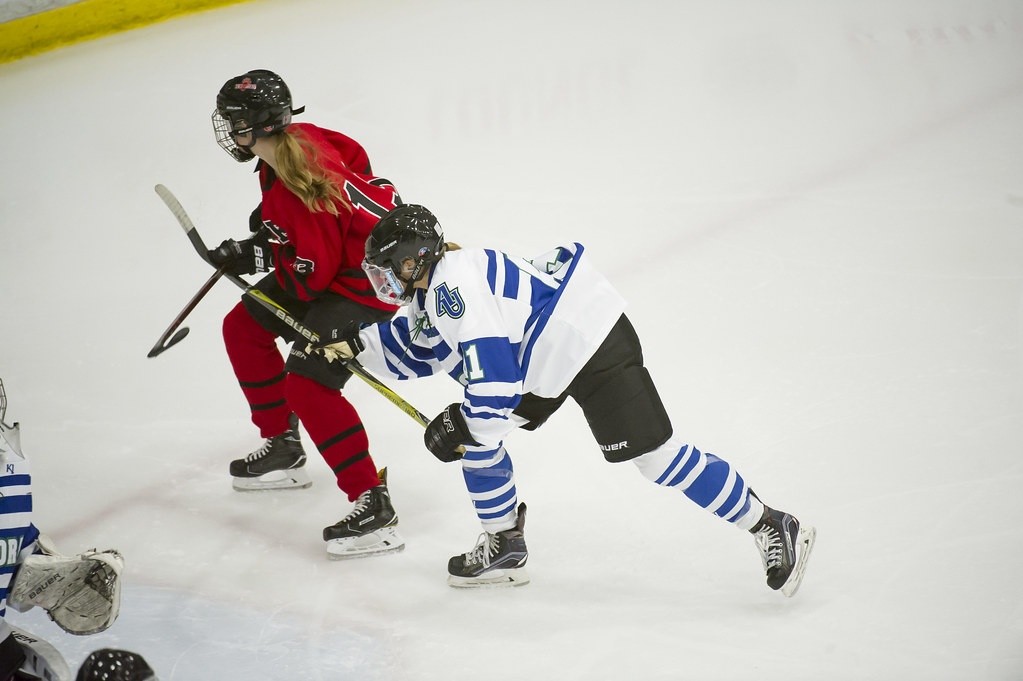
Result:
[208,68,411,561]
[304,203,817,599]
[0,376,159,681]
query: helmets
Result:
[361,203,446,307]
[76,649,154,681]
[212,69,305,163]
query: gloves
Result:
[207,234,276,276]
[425,403,484,462]
[304,319,365,376]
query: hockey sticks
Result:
[152,180,469,455]
[144,271,220,361]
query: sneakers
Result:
[323,467,405,560]
[746,487,817,599]
[230,413,313,493]
[447,502,530,589]
